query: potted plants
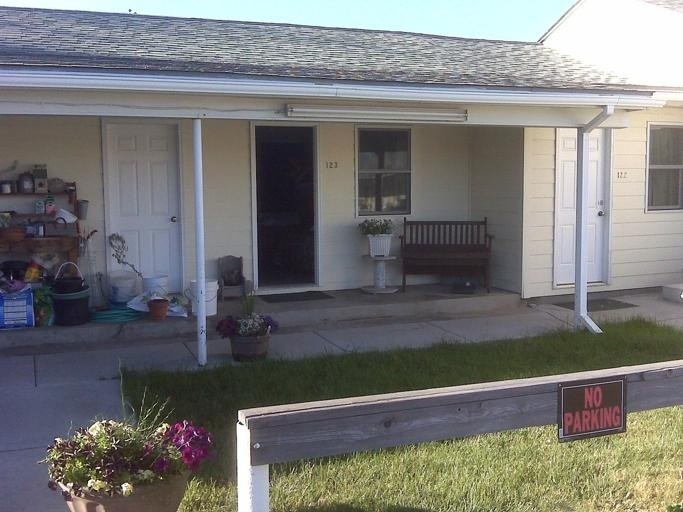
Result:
[359,216,394,257]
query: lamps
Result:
[284,105,468,125]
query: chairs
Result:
[216,254,246,303]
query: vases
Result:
[230,336,269,361]
[56,476,186,511]
[147,299,168,319]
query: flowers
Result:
[36,357,216,501]
[215,312,279,339]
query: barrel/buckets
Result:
[142,275,169,299]
[52,285,91,324]
[190,279,219,316]
[52,261,84,293]
[110,270,136,304]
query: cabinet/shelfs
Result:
[0,181,78,275]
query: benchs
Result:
[398,217,494,293]
